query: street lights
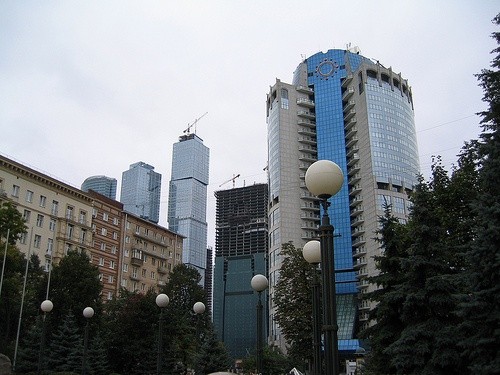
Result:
[37,300,54,375]
[250,274,268,375]
[82,307,95,375]
[302,239,323,374]
[304,159,344,375]
[193,302,206,375]
[303,240,323,375]
[155,294,170,375]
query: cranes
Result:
[182,111,208,135]
[218,173,241,189]
